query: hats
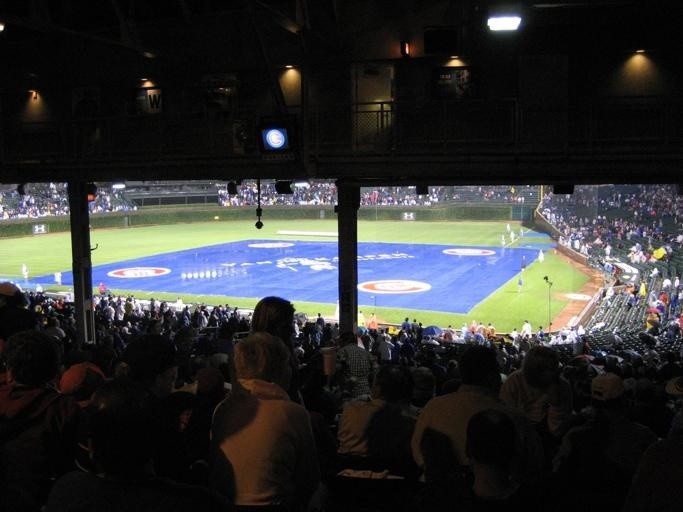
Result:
[56,361,116,397]
[591,371,637,402]
[1,281,26,296]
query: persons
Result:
[539,249,545,263]
[518,277,522,291]
[361,187,444,208]
[214,181,338,206]
[521,256,527,270]
[453,186,525,203]
[0,280,683,511]
[55,271,62,286]
[500,223,524,246]
[540,184,683,278]
[0,183,138,220]
[22,264,28,278]
